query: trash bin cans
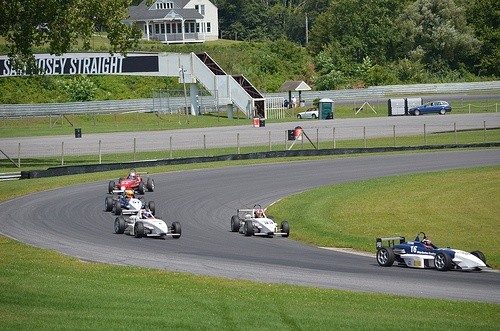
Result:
[300,100,306,107]
[388,97,406,116]
[405,97,423,116]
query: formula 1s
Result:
[230,207,290,237]
[104,168,182,239]
[376,232,493,271]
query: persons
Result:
[125,190,135,200]
[290,95,296,108]
[255,209,264,218]
[422,236,432,248]
[129,173,136,180]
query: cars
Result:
[409,101,451,115]
[298,108,318,118]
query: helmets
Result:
[256,209,264,217]
[423,239,429,244]
[143,209,151,216]
[126,190,133,198]
[130,173,135,179]
